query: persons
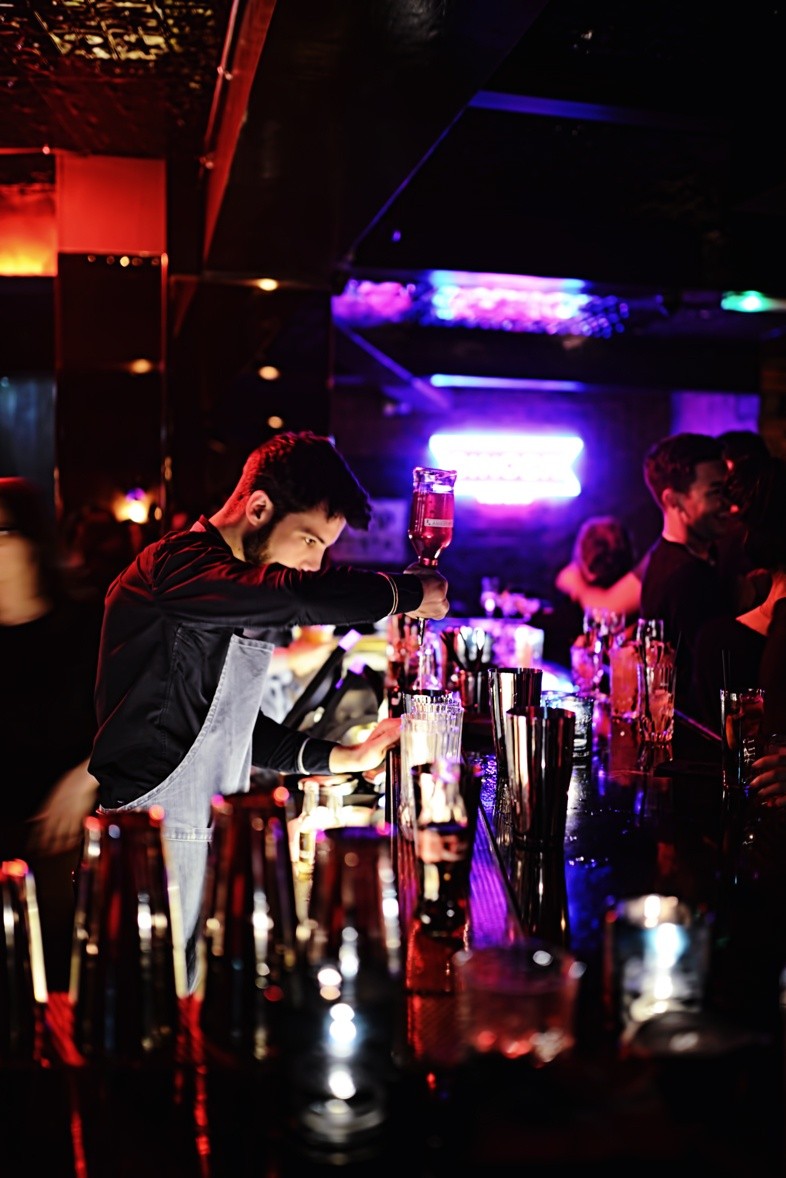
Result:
[0,474,98,996]
[552,427,785,816]
[92,430,449,1002]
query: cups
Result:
[544,604,673,769]
[485,667,543,768]
[719,690,764,802]
[505,705,576,847]
[605,875,712,1067]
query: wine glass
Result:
[736,736,775,847]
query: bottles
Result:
[406,467,458,641]
